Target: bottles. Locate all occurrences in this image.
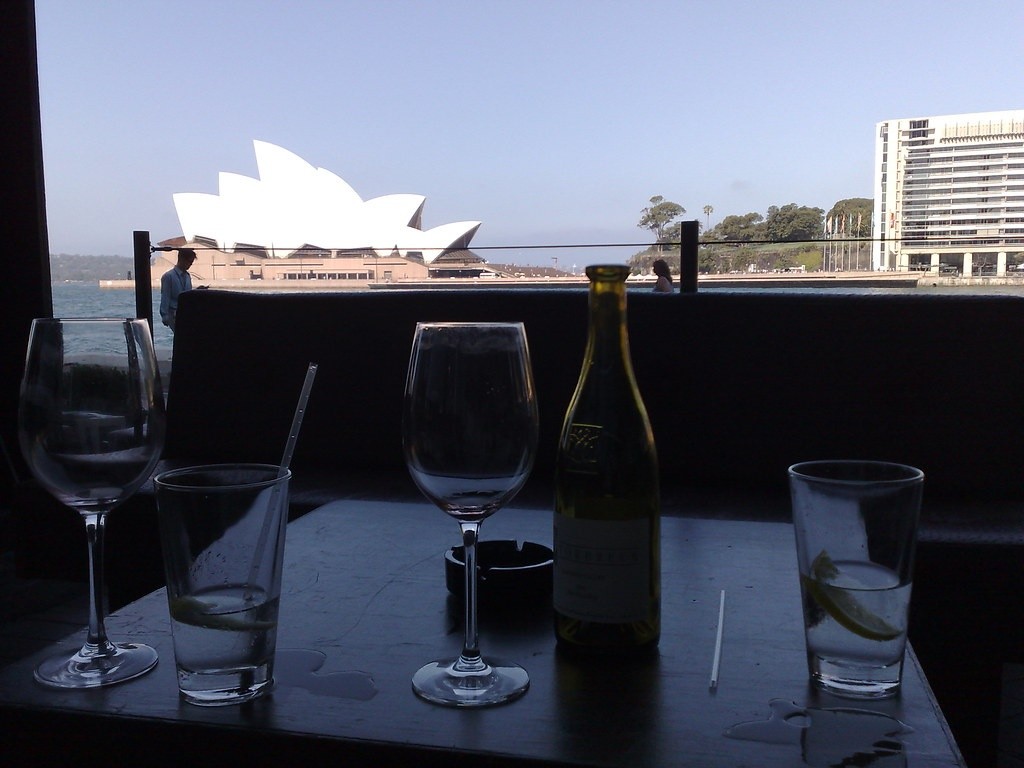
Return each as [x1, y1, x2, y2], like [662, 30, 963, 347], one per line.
[556, 264, 663, 664]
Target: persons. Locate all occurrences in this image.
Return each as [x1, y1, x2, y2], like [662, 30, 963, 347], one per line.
[652, 259, 675, 295]
[159, 250, 209, 336]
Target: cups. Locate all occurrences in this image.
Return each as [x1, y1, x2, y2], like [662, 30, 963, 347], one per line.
[788, 459, 928, 700]
[154, 461, 293, 707]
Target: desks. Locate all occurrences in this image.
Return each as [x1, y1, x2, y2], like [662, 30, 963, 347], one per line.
[0, 497, 967, 767]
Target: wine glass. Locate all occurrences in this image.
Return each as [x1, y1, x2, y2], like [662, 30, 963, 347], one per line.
[401, 320, 540, 709]
[21, 317, 166, 691]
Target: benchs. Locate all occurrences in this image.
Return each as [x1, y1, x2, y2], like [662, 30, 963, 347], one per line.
[106, 294, 1023, 665]
